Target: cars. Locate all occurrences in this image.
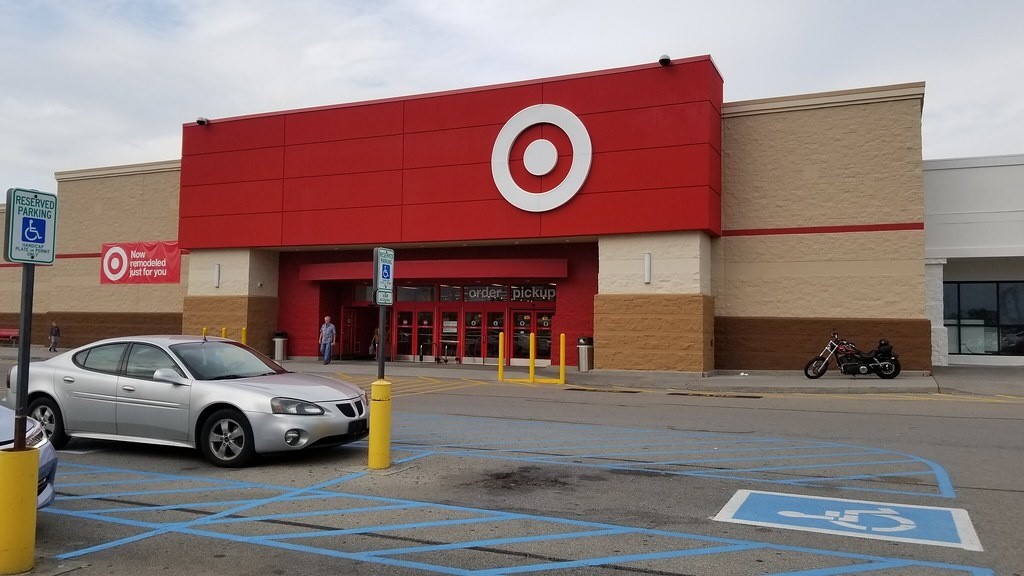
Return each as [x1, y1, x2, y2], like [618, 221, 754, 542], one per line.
[0, 404, 58, 512]
[5, 333, 372, 469]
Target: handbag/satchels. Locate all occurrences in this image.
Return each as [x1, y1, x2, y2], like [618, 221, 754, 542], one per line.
[369, 344, 374, 355]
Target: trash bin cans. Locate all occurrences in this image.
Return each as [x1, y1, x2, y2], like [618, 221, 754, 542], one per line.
[272, 331, 288, 361]
[577, 336, 594, 372]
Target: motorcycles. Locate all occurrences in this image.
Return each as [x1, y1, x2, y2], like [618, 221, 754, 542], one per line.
[804, 326, 902, 380]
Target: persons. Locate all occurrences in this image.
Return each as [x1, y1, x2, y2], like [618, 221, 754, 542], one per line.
[319, 316, 336, 365]
[49, 321, 60, 352]
[371, 327, 379, 359]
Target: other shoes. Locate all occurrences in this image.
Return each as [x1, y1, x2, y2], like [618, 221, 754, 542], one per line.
[324, 356, 332, 365]
[53, 349, 57, 352]
[49, 349, 51, 352]
